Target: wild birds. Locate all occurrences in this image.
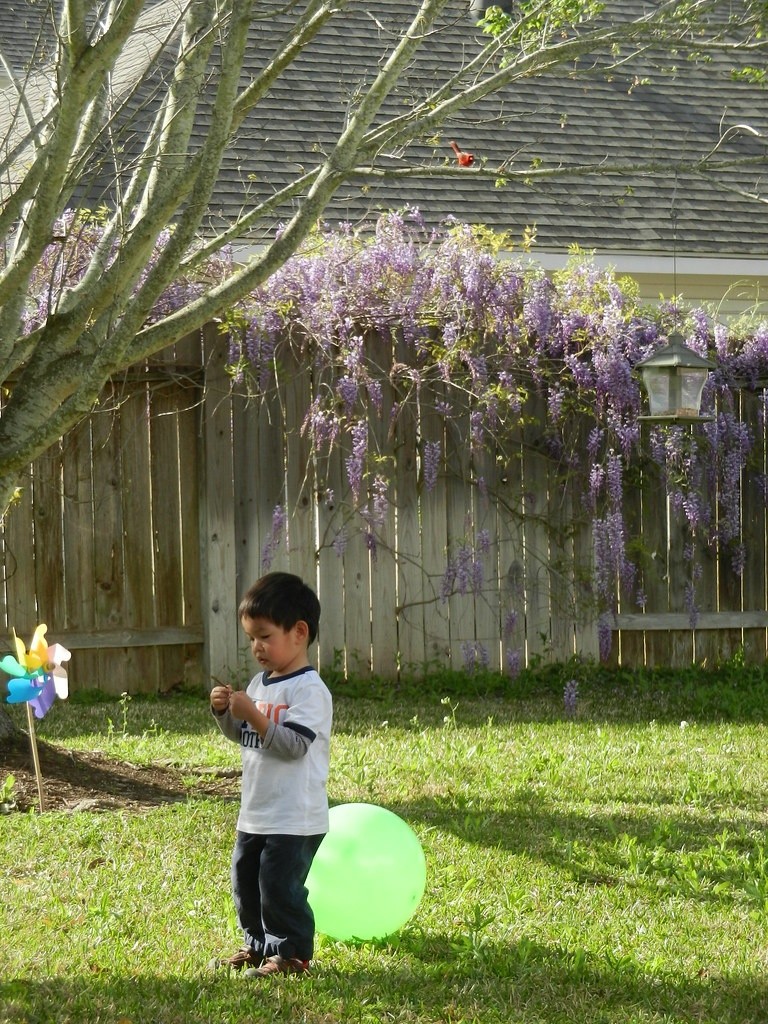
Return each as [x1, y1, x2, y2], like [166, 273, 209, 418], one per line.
[447, 141, 474, 168]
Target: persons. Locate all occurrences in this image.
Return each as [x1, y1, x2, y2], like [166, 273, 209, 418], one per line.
[209, 571, 336, 979]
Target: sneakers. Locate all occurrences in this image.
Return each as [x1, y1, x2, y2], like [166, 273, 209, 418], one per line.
[218, 944, 268, 971]
[245, 954, 308, 978]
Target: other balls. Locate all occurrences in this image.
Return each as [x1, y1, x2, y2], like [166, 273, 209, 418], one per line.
[307, 802, 428, 942]
